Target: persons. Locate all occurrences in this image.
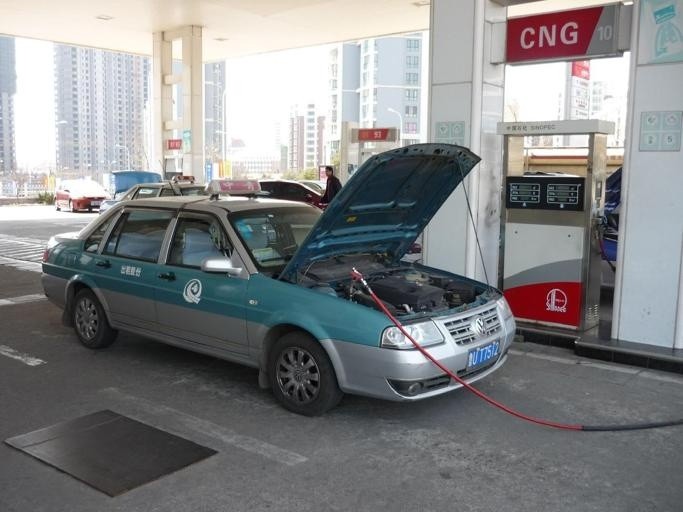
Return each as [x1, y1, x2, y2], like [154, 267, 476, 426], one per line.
[320, 166, 342, 203]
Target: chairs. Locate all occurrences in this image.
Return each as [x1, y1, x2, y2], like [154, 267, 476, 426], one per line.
[242, 230, 267, 252]
[169, 223, 223, 269]
